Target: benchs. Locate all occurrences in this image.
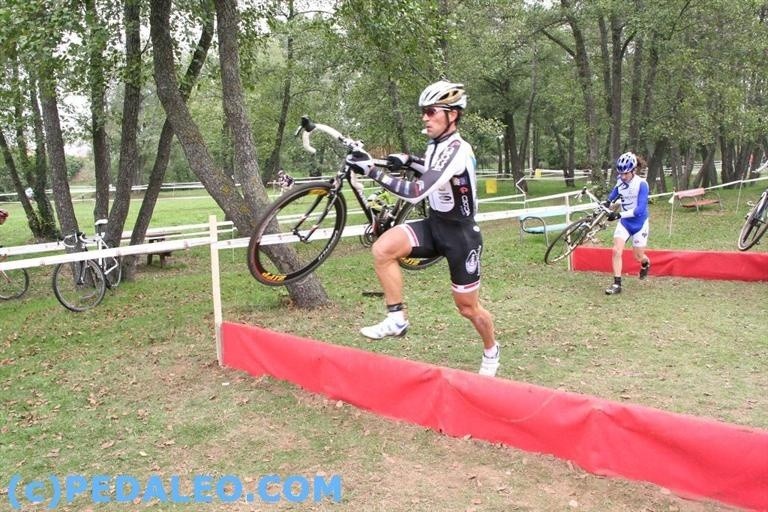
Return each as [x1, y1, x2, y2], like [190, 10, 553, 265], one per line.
[676, 185, 725, 217]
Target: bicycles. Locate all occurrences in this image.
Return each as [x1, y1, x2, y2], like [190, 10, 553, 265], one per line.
[245, 115, 449, 287]
[738, 161, 768, 251]
[54, 219, 124, 312]
[541, 186, 620, 266]
[0, 246, 29, 302]
[273, 182, 303, 205]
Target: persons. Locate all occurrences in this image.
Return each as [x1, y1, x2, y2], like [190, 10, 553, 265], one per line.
[605, 150, 652, 296]
[277, 170, 295, 195]
[344, 77, 503, 378]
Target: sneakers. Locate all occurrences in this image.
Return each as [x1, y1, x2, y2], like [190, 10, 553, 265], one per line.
[605, 282, 621, 294]
[359, 310, 408, 341]
[477, 344, 501, 378]
[638, 260, 650, 280]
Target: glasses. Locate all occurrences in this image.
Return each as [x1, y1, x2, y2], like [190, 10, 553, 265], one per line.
[421, 106, 452, 115]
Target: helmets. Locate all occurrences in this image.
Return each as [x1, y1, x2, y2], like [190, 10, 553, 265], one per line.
[616, 151, 638, 173]
[278, 169, 284, 176]
[417, 79, 466, 109]
